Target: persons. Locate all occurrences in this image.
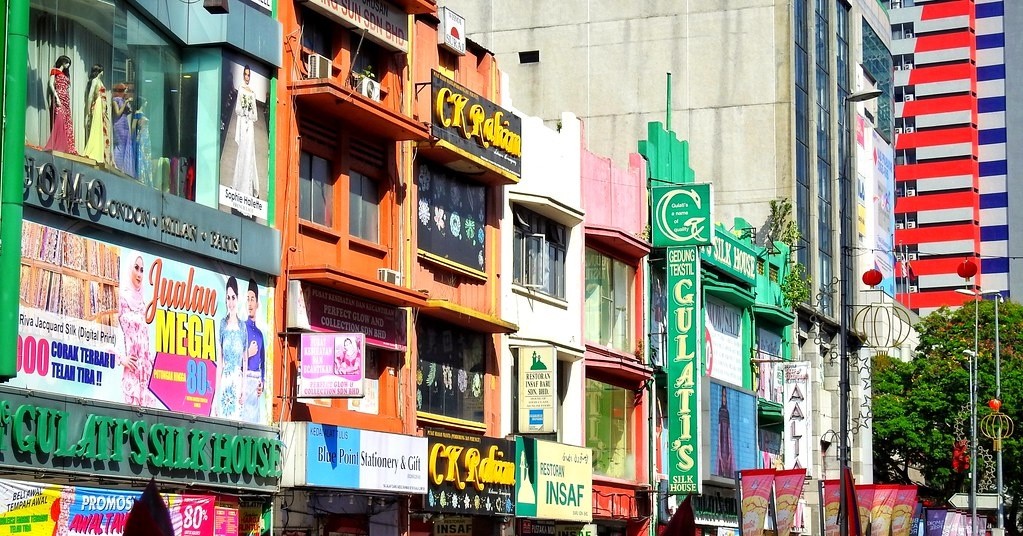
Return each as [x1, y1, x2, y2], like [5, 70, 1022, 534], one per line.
[131, 95, 154, 187]
[112, 82, 136, 176]
[716, 386, 737, 478]
[336, 338, 360, 376]
[213, 276, 247, 421]
[48, 54, 76, 154]
[234, 64, 259, 198]
[86, 64, 114, 164]
[104, 253, 161, 409]
[242, 278, 266, 425]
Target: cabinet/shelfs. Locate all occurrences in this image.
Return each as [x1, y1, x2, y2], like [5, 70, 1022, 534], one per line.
[17, 217, 120, 329]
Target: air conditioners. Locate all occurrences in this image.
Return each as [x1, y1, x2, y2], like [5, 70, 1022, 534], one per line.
[896, 253, 917, 261]
[907, 190, 916, 197]
[308, 54, 333, 79]
[906, 127, 915, 133]
[908, 286, 918, 293]
[895, 66, 901, 71]
[895, 128, 902, 134]
[895, 223, 905, 230]
[905, 94, 914, 102]
[907, 221, 916, 229]
[906, 33, 913, 39]
[377, 267, 403, 287]
[904, 64, 913, 70]
[356, 75, 381, 103]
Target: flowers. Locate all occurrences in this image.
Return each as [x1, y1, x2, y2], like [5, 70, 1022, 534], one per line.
[240, 93, 255, 115]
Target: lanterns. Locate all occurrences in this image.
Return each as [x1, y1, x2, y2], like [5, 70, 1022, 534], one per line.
[957, 260, 978, 281]
[862, 269, 882, 289]
[989, 399, 1001, 411]
